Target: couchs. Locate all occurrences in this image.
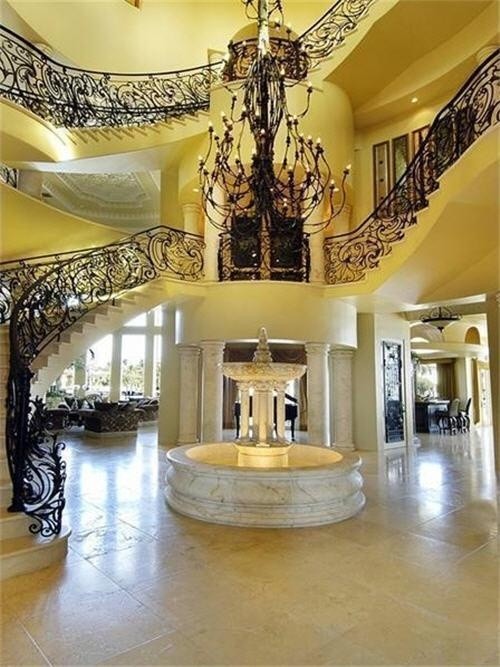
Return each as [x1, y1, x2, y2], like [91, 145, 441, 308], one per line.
[45, 390, 159, 439]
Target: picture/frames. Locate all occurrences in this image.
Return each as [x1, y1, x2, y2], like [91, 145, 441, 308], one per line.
[369, 101, 476, 221]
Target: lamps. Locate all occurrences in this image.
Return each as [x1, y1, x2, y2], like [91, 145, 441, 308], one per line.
[184, 1, 353, 239]
[418, 306, 463, 334]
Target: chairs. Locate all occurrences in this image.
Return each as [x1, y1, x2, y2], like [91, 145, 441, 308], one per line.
[435, 398, 471, 435]
